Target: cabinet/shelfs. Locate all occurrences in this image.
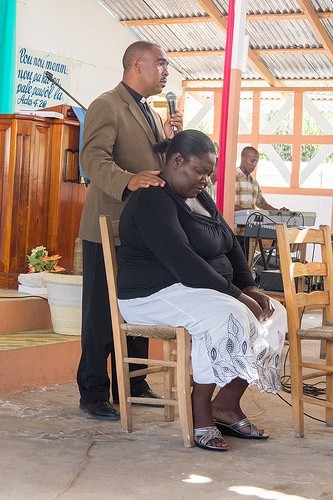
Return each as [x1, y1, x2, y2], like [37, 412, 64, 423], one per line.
[0, 114, 91, 288]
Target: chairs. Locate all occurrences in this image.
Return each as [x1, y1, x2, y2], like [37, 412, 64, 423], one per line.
[99, 214, 196, 448]
[275, 223, 333, 439]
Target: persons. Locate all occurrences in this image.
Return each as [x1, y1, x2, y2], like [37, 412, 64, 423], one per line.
[76, 41, 183, 421]
[202, 147, 283, 264]
[117, 129, 287, 451]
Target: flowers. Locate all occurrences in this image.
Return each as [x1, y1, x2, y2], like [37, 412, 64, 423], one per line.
[27, 245, 66, 274]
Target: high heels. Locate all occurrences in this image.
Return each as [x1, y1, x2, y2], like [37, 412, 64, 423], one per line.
[214, 418, 270, 440]
[192, 425, 230, 452]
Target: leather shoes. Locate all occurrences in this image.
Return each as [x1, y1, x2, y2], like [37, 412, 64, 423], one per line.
[112, 389, 166, 408]
[79, 400, 121, 421]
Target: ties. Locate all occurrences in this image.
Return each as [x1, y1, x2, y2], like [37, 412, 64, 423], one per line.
[142, 103, 161, 141]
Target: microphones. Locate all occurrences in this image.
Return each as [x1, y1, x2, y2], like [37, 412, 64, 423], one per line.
[166, 91, 179, 131]
